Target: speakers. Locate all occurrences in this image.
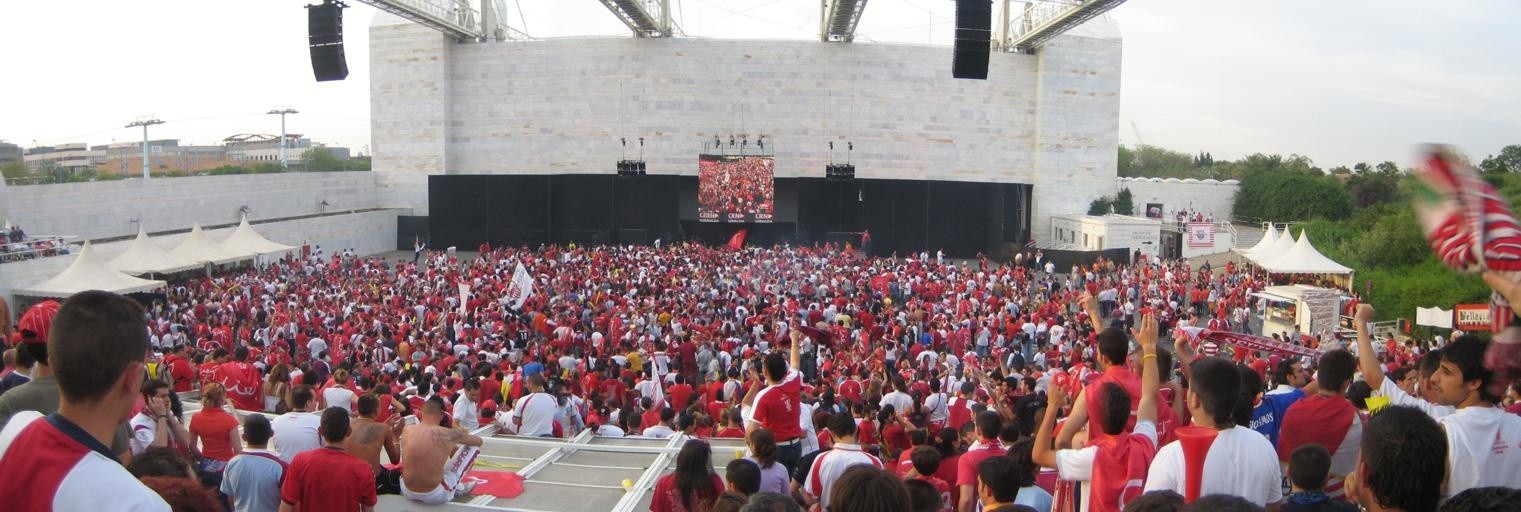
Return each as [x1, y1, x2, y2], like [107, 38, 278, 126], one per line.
[308, 5, 349, 82]
[952, 0, 993, 78]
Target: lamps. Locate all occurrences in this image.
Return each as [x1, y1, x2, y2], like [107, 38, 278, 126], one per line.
[639, 137, 644, 161]
[848, 140, 853, 164]
[714, 133, 766, 157]
[621, 138, 626, 160]
[829, 141, 834, 165]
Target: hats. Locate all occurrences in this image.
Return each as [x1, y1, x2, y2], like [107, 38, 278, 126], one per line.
[20, 300, 58, 345]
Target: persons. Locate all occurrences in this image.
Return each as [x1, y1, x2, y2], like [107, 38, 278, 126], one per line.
[698, 158, 774, 207]
[2, 209, 1520, 510]
